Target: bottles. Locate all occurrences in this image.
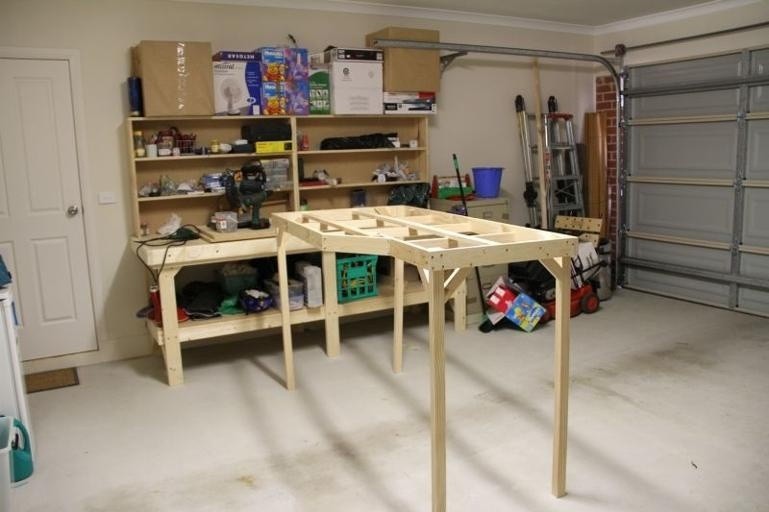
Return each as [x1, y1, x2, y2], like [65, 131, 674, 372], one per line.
[134, 131, 147, 157]
[211, 136, 220, 153]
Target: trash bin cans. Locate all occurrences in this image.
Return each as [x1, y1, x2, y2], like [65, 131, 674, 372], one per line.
[594, 238, 613, 301]
[0, 415, 14, 512]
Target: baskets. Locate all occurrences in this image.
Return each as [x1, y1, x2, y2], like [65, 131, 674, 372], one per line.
[335, 253, 382, 306]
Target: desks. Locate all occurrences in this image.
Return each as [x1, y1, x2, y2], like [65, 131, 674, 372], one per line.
[132, 238, 468, 387]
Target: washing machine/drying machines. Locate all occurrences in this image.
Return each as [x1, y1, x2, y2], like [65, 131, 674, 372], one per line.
[430, 173, 509, 320]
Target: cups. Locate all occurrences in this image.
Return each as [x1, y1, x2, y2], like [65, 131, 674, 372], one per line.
[146, 144, 158, 157]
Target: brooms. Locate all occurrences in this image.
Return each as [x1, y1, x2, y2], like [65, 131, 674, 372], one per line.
[452, 152, 509, 332]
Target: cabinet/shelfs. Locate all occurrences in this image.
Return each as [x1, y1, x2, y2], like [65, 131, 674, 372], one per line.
[126, 114, 430, 238]
[432, 196, 512, 318]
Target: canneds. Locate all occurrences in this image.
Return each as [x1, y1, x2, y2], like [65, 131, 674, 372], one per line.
[210, 140, 219, 153]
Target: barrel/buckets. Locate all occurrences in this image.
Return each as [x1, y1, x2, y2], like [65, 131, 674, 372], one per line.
[472, 167, 506, 197]
[293, 260, 322, 307]
[0, 414, 35, 482]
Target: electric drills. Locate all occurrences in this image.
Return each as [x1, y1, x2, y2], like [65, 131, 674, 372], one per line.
[243, 190, 274, 229]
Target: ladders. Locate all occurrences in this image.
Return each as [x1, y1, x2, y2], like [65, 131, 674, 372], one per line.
[514, 94, 587, 232]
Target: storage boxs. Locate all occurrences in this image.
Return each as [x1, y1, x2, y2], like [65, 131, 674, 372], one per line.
[308, 46, 384, 114]
[306, 61, 331, 113]
[484, 275, 546, 332]
[213, 49, 262, 115]
[130, 26, 214, 117]
[364, 27, 440, 112]
[256, 45, 309, 115]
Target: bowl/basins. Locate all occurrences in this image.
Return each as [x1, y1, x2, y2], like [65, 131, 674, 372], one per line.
[238, 296, 273, 313]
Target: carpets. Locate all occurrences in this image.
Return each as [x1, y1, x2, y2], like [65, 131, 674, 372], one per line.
[24, 366, 79, 394]
